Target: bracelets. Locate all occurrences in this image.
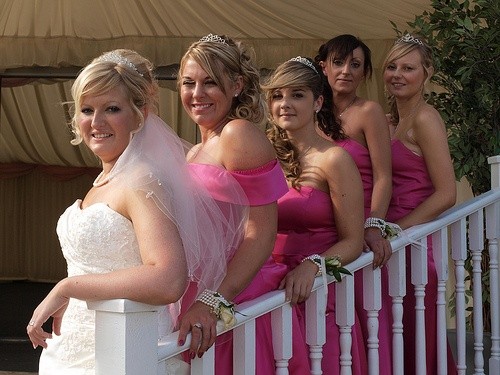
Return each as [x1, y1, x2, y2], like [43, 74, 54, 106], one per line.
[363, 217, 402, 238]
[300, 253, 353, 284]
[195, 287, 249, 330]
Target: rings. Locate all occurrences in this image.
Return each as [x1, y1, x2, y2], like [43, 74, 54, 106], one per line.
[192, 323, 203, 330]
[29, 320, 35, 326]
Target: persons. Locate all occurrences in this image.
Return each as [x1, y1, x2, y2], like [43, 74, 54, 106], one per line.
[24, 47, 249, 375]
[381, 33, 458, 375]
[171, 31, 308, 375]
[263, 57, 368, 375]
[315, 34, 393, 375]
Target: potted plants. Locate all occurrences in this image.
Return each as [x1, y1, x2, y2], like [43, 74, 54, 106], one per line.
[390, 0, 500, 375]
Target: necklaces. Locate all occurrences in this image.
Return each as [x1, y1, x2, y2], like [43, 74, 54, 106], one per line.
[91, 152, 148, 189]
[336, 95, 359, 120]
[297, 135, 319, 159]
